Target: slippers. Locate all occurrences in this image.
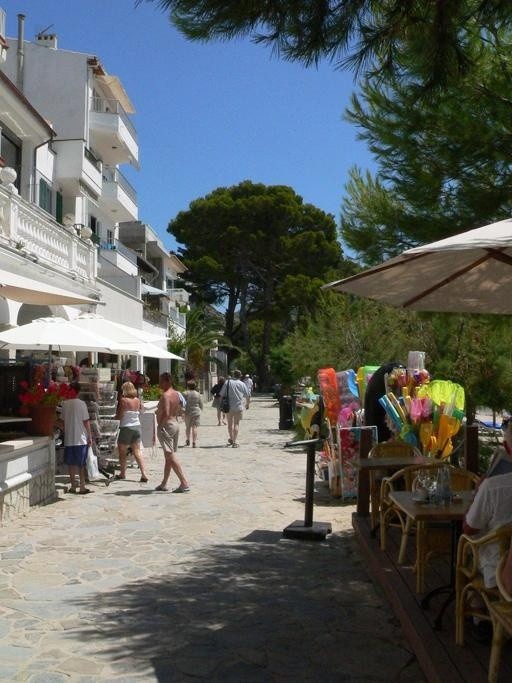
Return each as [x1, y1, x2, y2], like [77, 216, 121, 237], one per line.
[64, 474, 193, 500]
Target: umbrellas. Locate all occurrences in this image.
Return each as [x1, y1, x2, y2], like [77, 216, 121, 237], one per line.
[319, 217, 512, 315]
[0, 310, 186, 366]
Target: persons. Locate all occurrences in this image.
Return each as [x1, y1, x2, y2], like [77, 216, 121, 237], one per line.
[181, 379, 204, 448]
[154, 372, 190, 493]
[210, 369, 257, 448]
[461, 417, 512, 614]
[60, 381, 94, 494]
[112, 381, 149, 482]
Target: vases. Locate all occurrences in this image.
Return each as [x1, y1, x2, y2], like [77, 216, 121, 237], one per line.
[26, 406, 58, 436]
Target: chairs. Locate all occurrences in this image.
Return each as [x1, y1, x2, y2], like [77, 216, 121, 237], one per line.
[365, 439, 511, 682]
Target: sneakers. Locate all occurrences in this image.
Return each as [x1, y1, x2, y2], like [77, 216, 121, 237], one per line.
[227, 438, 240, 448]
[185, 437, 198, 449]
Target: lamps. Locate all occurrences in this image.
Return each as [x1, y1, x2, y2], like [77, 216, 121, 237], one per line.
[73, 222, 93, 239]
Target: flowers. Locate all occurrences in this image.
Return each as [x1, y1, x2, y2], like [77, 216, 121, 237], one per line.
[18, 379, 79, 416]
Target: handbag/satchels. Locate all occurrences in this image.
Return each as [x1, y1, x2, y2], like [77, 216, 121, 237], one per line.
[218, 399, 232, 414]
[85, 447, 104, 482]
